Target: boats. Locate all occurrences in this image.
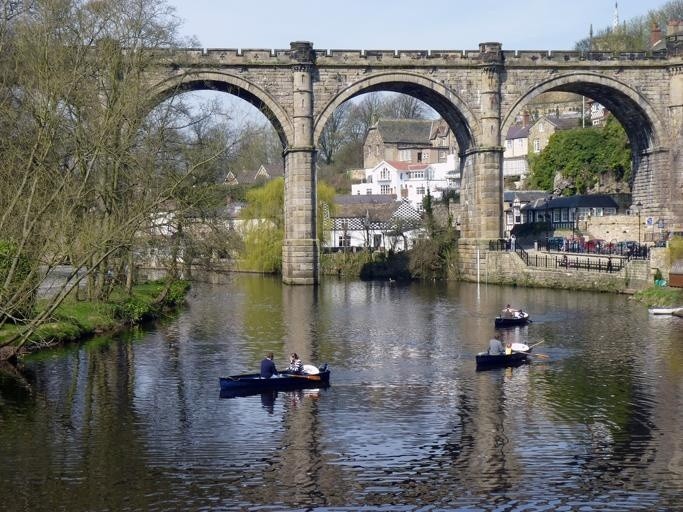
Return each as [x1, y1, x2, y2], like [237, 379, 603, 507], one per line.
[475, 342, 531, 368]
[218, 363, 331, 392]
[495, 312, 529, 329]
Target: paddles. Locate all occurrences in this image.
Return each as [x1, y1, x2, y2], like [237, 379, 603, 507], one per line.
[514, 350, 549, 360]
[279, 373, 320, 381]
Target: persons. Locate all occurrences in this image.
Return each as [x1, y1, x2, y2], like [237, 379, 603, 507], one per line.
[260, 352, 287, 378]
[260, 391, 278, 416]
[510, 234, 516, 250]
[486, 333, 505, 355]
[559, 233, 586, 253]
[285, 390, 303, 408]
[501, 304, 521, 319]
[288, 352, 303, 375]
[489, 367, 505, 388]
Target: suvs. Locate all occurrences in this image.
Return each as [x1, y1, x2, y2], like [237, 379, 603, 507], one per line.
[546, 236, 564, 251]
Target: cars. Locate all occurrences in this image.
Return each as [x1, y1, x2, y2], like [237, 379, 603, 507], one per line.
[581, 239, 642, 257]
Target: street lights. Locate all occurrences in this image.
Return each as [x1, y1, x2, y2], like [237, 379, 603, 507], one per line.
[636, 201, 643, 257]
